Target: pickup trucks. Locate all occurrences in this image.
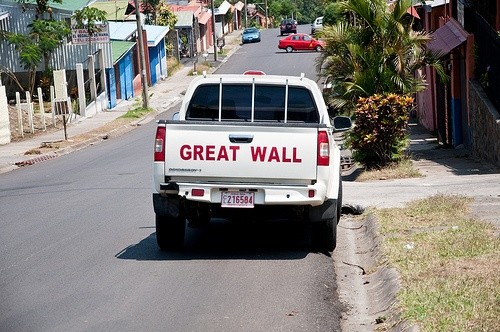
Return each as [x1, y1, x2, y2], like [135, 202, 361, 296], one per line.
[152, 70, 352, 253]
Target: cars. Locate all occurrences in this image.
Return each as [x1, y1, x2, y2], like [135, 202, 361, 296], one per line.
[310, 16, 324, 35]
[277, 33, 326, 53]
[241, 27, 261, 42]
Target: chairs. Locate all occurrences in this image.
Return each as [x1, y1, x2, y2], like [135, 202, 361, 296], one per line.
[272, 101, 307, 122]
[292, 36, 304, 40]
[208, 99, 238, 119]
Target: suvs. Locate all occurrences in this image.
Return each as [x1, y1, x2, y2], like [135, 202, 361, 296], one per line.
[279, 18, 301, 34]
